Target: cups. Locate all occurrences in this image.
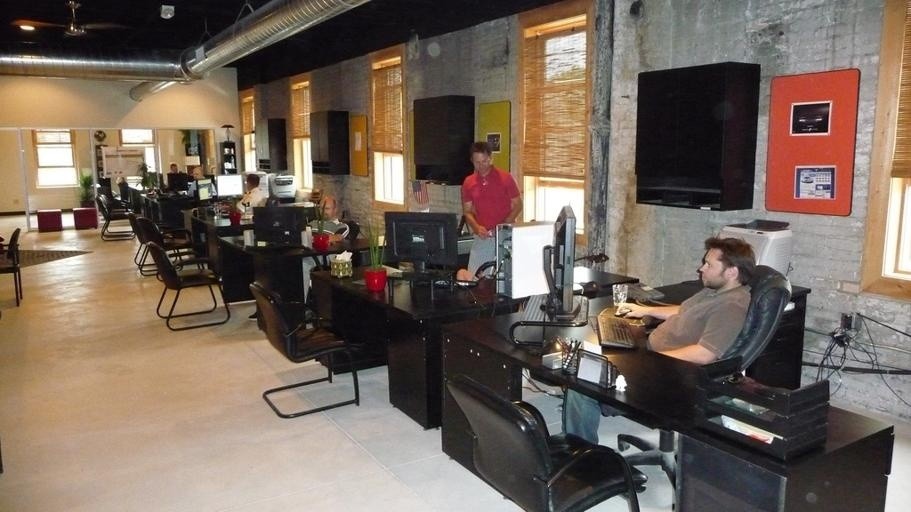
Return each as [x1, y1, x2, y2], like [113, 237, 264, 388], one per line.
[562, 349, 577, 375]
[612, 285, 628, 313]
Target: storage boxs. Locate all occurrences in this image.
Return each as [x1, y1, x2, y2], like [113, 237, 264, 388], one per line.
[36, 208, 63, 232]
[72, 207, 99, 230]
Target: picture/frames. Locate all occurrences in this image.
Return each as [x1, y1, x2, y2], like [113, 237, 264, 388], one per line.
[789, 100, 832, 136]
[792, 163, 837, 201]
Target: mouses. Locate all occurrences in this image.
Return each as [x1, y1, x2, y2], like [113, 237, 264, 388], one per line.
[615, 308, 631, 317]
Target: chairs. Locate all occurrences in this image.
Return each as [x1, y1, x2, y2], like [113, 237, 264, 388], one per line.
[341, 218, 362, 243]
[615, 263, 791, 511]
[95, 192, 138, 242]
[145, 240, 232, 332]
[446, 371, 647, 511]
[0, 227, 25, 309]
[134, 216, 191, 277]
[95, 183, 136, 234]
[249, 277, 360, 419]
[128, 212, 178, 267]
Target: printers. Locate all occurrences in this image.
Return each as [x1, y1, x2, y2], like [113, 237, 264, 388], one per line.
[269, 174, 297, 198]
[717, 219, 794, 278]
[241, 171, 268, 196]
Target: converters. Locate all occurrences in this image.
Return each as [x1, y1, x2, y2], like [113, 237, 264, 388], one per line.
[841, 312, 851, 330]
[830, 331, 851, 347]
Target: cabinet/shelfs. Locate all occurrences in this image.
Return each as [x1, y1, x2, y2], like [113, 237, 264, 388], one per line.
[635, 59, 763, 212]
[219, 141, 238, 174]
[411, 95, 475, 188]
[308, 111, 351, 176]
[253, 117, 288, 173]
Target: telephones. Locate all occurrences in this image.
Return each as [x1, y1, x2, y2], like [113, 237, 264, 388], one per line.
[627, 282, 664, 303]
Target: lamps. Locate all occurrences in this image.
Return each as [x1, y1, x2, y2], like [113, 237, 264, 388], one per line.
[221, 124, 235, 141]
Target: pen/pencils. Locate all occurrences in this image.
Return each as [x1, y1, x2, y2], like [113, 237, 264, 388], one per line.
[556, 335, 581, 370]
[493, 260, 504, 277]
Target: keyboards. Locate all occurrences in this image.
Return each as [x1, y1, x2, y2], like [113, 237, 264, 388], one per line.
[387, 271, 403, 279]
[597, 315, 636, 350]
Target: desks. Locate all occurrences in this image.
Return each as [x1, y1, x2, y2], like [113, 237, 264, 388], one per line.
[212, 230, 376, 320]
[307, 257, 522, 433]
[178, 203, 254, 275]
[435, 285, 897, 512]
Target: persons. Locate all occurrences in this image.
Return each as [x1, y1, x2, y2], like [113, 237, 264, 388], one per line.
[460, 142, 523, 276]
[239, 175, 266, 219]
[562, 238, 757, 445]
[166, 163, 179, 186]
[247, 195, 350, 320]
[184, 167, 217, 231]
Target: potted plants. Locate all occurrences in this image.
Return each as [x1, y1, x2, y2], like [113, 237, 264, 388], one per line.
[306, 200, 329, 252]
[78, 173, 95, 208]
[229, 198, 241, 225]
[359, 218, 387, 293]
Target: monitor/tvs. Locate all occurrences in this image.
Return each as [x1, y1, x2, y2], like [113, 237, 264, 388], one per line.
[216, 174, 244, 203]
[543, 206, 581, 320]
[158, 172, 164, 192]
[167, 173, 188, 196]
[196, 178, 214, 208]
[384, 210, 458, 281]
[253, 206, 315, 250]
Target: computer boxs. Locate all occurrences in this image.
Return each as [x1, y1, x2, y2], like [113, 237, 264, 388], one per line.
[495, 220, 555, 300]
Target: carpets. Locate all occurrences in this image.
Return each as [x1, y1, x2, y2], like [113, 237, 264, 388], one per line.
[18, 249, 94, 268]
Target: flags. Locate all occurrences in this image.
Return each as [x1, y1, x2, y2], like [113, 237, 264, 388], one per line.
[412, 180, 429, 207]
